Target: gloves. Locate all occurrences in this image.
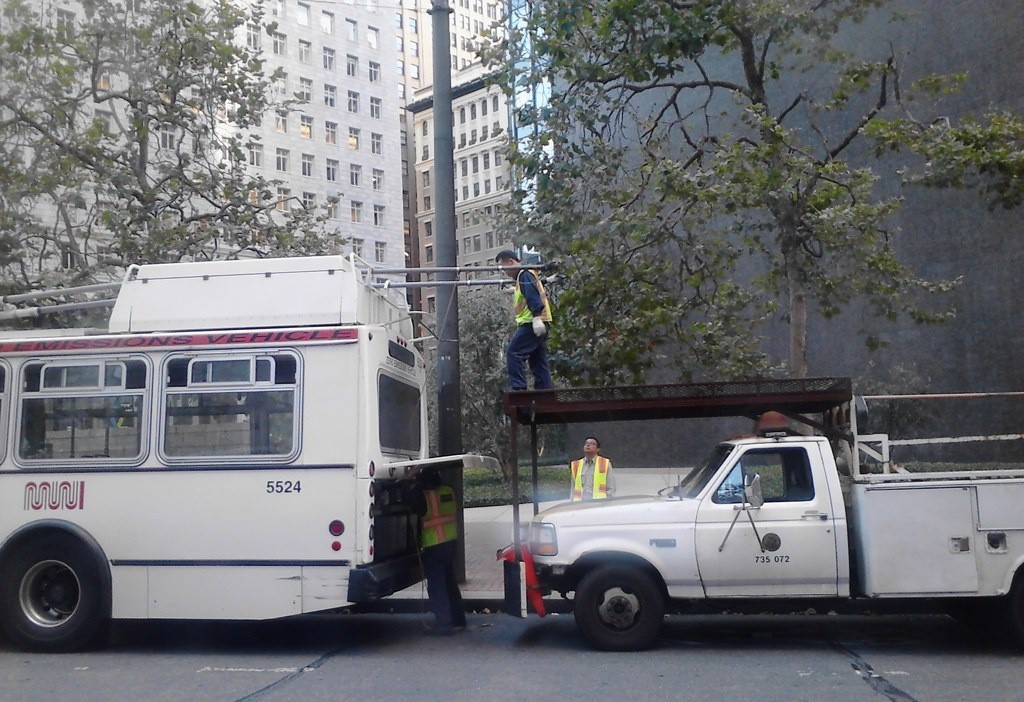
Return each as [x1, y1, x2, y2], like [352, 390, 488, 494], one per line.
[533, 317, 547, 336]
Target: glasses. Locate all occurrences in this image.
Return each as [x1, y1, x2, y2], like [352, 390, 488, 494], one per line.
[584, 442, 598, 447]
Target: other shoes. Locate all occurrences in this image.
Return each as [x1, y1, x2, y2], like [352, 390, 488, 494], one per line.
[428, 626, 463, 635]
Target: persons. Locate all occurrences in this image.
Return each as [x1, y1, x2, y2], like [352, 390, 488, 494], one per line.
[569, 438, 617, 501]
[494, 249, 561, 390]
[404, 463, 469, 637]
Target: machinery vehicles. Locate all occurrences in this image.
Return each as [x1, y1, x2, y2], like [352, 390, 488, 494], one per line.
[0, 256, 502, 646]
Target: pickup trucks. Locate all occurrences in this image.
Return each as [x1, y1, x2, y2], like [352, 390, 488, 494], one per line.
[521, 426, 1023, 655]
